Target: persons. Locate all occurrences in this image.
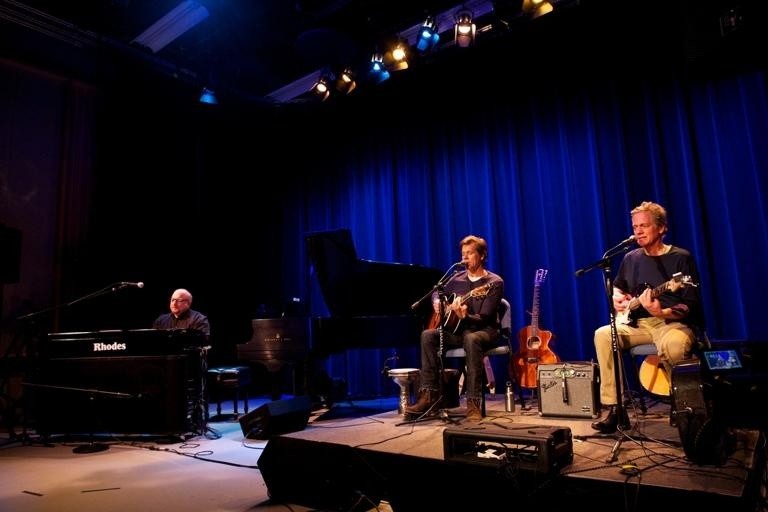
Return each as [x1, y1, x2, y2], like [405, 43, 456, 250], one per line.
[592, 201, 707, 433]
[153, 288, 211, 341]
[405, 235, 505, 421]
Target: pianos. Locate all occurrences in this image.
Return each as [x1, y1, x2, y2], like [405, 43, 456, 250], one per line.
[236, 228, 450, 409]
[24, 329, 210, 438]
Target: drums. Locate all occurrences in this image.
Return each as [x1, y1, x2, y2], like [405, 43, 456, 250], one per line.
[444, 370, 460, 408]
[388, 368, 420, 414]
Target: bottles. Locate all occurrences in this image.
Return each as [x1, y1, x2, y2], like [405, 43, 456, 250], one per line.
[504, 381, 515, 412]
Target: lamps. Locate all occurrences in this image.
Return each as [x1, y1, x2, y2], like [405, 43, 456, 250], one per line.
[453, 8, 478, 50]
[522, 0, 555, 20]
[309, 76, 333, 102]
[337, 63, 359, 94]
[417, 15, 440, 52]
[386, 37, 410, 71]
[369, 53, 390, 82]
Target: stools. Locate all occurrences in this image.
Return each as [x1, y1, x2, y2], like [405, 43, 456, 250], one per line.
[204, 365, 250, 420]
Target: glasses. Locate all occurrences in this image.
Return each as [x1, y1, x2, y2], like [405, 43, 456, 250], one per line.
[171, 298, 193, 303]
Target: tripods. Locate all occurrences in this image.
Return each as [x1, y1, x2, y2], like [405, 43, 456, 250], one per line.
[395, 311, 466, 427]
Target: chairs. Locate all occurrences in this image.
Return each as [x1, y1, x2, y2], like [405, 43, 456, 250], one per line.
[446, 298, 526, 417]
[627, 330, 711, 415]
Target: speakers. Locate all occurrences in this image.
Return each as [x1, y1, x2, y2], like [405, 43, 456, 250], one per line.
[237, 398, 311, 439]
[536, 362, 600, 418]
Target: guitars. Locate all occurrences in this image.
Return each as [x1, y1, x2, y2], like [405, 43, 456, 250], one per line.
[429, 281, 497, 334]
[508, 269, 561, 401]
[639, 355, 673, 400]
[613, 272, 699, 327]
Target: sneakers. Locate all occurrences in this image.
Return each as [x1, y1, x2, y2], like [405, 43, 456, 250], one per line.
[465, 396, 485, 422]
[404, 391, 440, 414]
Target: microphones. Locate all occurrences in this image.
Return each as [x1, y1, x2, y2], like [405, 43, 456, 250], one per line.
[616, 234, 638, 249]
[120, 281, 145, 289]
[455, 261, 467, 266]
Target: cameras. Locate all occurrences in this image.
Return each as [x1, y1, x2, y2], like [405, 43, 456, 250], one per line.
[570, 271, 682, 465]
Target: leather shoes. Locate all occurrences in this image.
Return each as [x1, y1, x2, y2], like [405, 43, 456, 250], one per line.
[591, 405, 632, 432]
